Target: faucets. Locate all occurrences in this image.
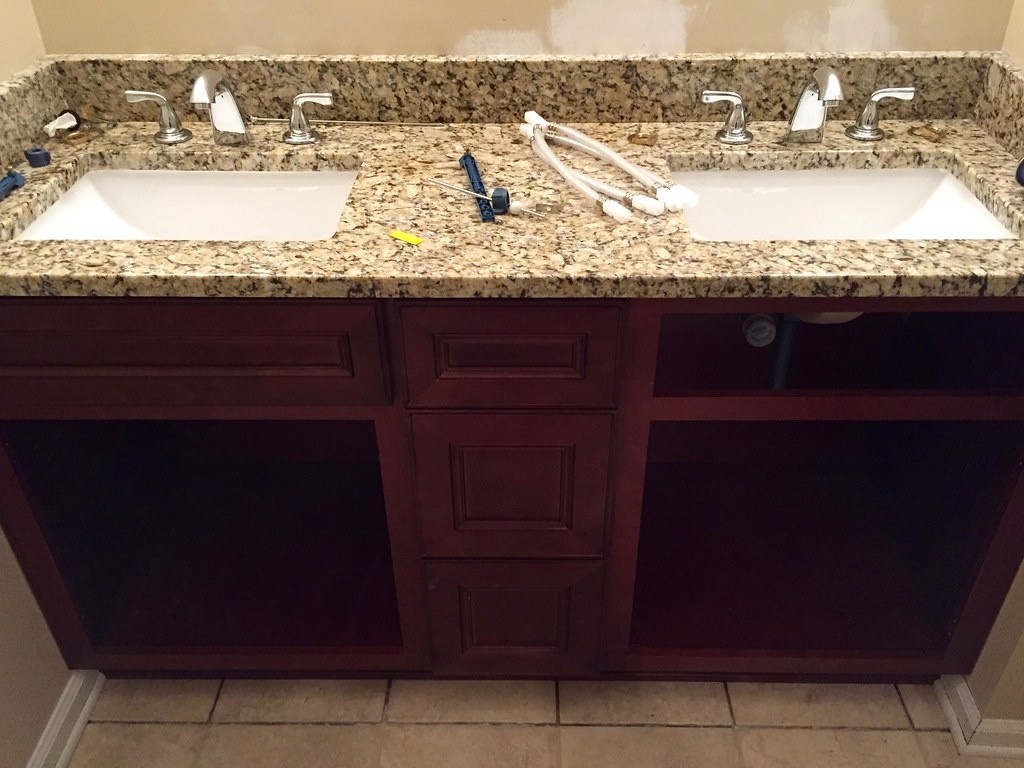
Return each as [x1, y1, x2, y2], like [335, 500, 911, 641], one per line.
[189, 69, 253, 146]
[784, 66, 844, 143]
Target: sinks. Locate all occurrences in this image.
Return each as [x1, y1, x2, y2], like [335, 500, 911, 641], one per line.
[3, 150, 364, 241]
[667, 150, 1024, 241]
